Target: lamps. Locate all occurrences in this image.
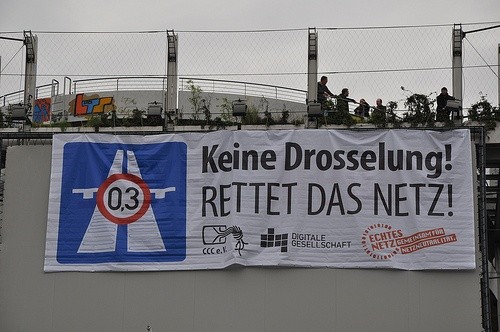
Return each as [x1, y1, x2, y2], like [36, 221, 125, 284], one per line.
[446, 99, 463, 121]
[147, 101, 162, 126]
[306, 103, 324, 127]
[9, 103, 32, 126]
[232, 99, 248, 129]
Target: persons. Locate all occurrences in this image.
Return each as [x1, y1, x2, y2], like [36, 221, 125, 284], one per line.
[354, 99, 370, 118]
[336, 87, 356, 113]
[317, 76, 336, 104]
[373, 97, 386, 115]
[437, 86, 456, 116]
